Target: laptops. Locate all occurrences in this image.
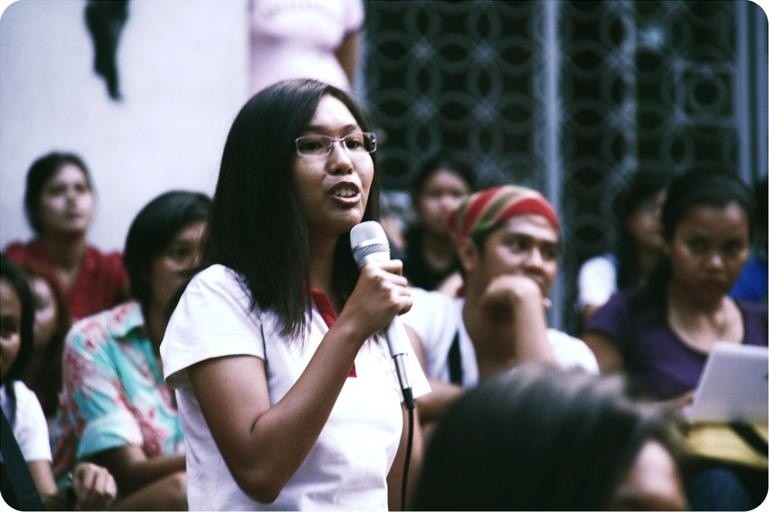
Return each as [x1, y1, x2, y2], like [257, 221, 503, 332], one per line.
[680, 340, 768, 424]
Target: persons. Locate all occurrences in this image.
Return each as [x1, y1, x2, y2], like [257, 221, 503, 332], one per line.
[5, 151, 132, 323]
[401, 157, 476, 291]
[249, 0, 367, 102]
[0, 252, 117, 510]
[20, 258, 74, 432]
[400, 185, 599, 443]
[159, 78, 431, 511]
[412, 356, 692, 511]
[51, 188, 212, 511]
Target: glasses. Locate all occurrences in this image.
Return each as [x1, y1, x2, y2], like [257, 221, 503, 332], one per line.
[294, 131, 377, 158]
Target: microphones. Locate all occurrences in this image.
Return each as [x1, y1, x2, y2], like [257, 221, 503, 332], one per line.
[350, 221, 414, 409]
